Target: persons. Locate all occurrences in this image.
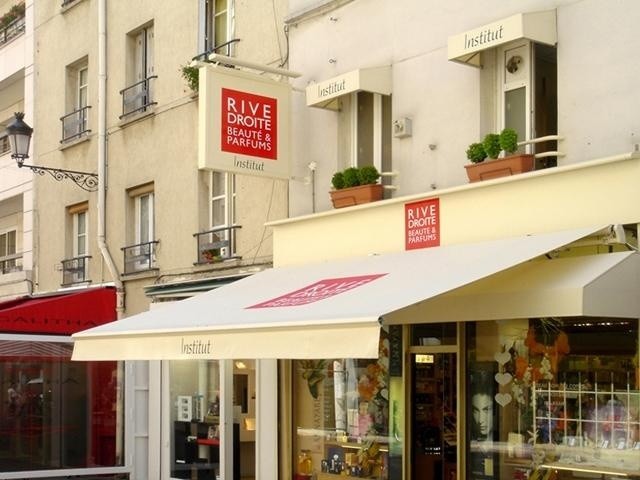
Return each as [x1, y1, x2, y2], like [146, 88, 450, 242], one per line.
[466, 381, 495, 474]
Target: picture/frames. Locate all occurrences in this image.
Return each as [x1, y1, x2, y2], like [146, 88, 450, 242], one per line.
[466, 360, 500, 479]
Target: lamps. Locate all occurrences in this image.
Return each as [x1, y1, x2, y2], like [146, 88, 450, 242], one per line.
[6, 112, 101, 194]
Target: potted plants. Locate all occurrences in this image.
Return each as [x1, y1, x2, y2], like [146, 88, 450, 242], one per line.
[464, 128, 535, 183]
[328, 164, 384, 209]
